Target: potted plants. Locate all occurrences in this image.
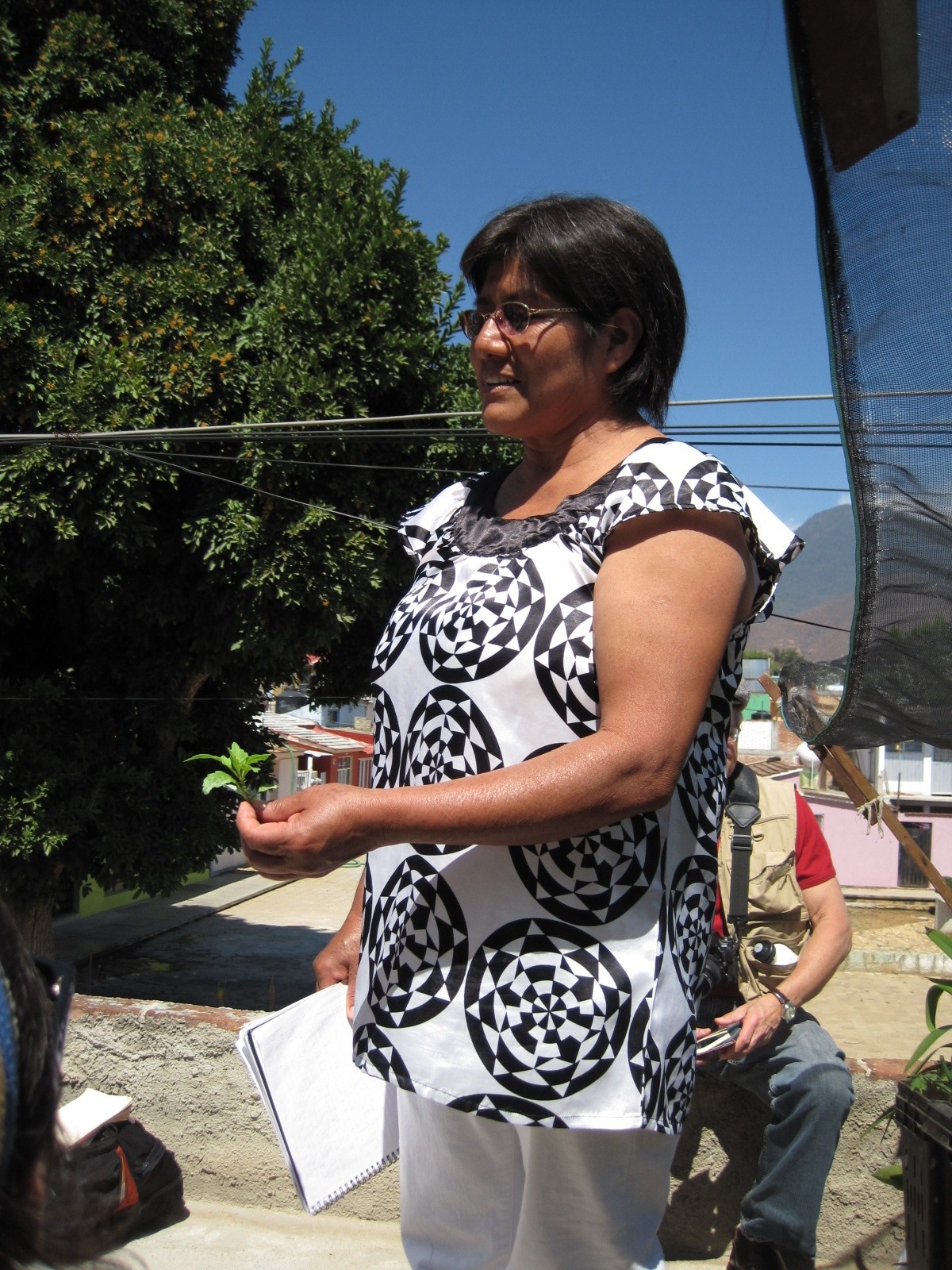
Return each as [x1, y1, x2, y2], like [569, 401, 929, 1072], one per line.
[856, 922, 952, 1270]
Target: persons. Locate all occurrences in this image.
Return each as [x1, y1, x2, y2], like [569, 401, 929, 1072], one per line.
[234, 194, 803, 1270]
[693, 680, 855, 1270]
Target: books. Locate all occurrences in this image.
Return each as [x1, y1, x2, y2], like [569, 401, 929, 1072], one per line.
[234, 977, 401, 1215]
[696, 1020, 740, 1057]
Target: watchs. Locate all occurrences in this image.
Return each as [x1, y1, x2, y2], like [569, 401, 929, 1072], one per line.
[768, 987, 798, 1024]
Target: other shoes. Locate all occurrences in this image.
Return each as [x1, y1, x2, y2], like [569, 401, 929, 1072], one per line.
[727, 1225, 814, 1270]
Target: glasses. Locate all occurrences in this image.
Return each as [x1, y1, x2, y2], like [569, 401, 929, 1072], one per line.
[457, 303, 586, 339]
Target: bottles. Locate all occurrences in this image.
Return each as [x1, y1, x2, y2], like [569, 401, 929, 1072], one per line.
[753, 940, 798, 965]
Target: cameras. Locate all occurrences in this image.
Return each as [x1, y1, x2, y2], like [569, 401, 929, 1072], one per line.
[700, 935, 738, 996]
